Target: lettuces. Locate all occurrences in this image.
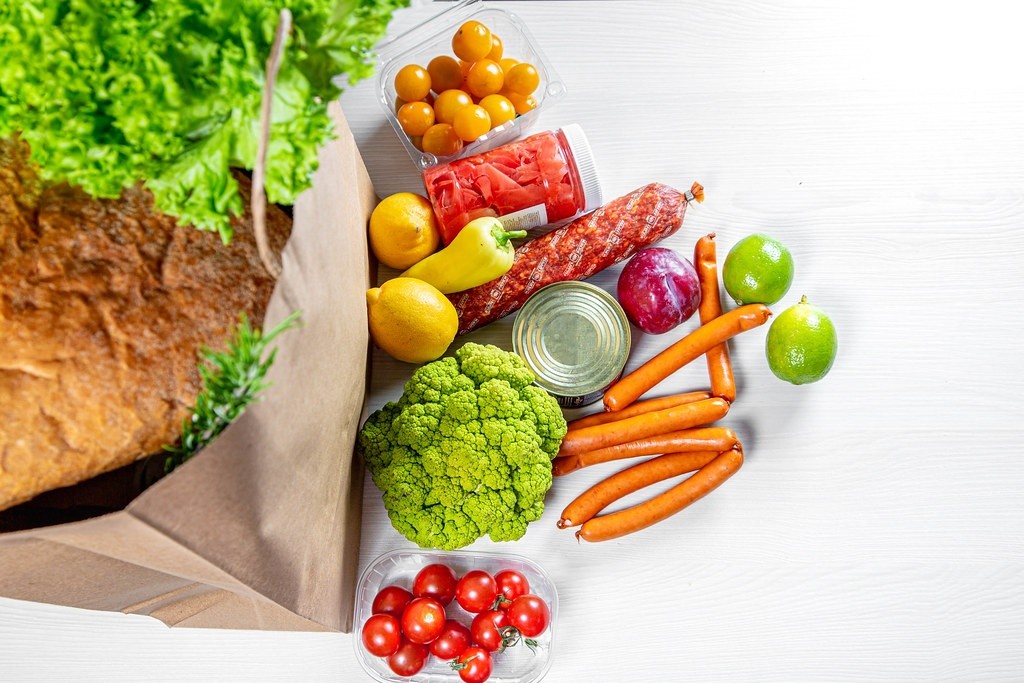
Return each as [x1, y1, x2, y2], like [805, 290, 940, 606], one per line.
[0, 2, 420, 247]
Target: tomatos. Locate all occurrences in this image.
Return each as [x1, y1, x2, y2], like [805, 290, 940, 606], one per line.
[365, 566, 553, 683]
[395, 21, 539, 157]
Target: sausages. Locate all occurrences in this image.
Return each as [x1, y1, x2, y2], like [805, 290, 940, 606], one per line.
[546, 230, 772, 544]
[444, 181, 713, 338]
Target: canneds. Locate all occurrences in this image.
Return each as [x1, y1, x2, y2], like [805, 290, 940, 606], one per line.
[423, 122, 612, 249]
[510, 279, 635, 410]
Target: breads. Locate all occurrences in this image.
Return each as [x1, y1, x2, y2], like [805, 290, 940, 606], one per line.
[0, 128, 294, 512]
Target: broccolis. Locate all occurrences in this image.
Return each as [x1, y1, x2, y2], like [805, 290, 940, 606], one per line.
[357, 340, 567, 551]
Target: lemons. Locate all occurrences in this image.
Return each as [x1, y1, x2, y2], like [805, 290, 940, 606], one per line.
[367, 189, 459, 367]
[725, 236, 838, 387]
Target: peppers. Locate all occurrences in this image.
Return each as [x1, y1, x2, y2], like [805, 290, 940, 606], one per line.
[395, 214, 533, 297]
